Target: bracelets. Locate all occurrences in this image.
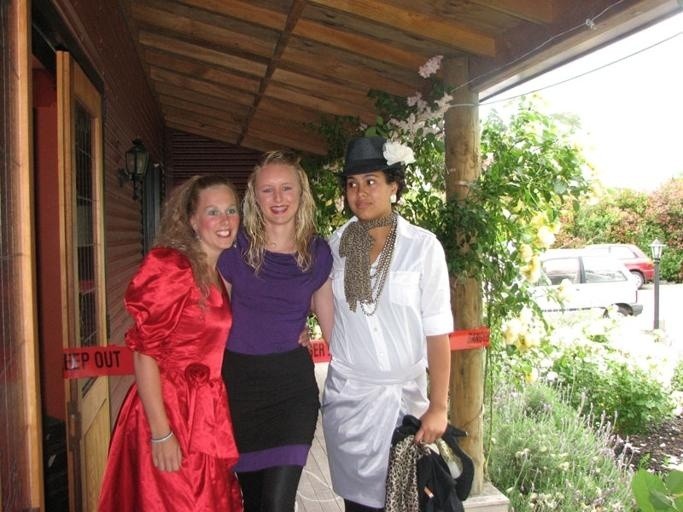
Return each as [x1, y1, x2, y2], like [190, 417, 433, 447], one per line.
[150, 431, 175, 444]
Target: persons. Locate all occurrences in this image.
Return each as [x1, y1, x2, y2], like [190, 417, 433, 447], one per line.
[215, 149, 334, 512]
[312, 137, 454, 512]
[98, 173, 245, 512]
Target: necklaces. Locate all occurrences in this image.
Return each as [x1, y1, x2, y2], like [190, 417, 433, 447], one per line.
[360, 214, 399, 317]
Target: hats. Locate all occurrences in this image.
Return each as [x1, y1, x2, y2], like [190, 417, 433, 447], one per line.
[332, 134, 403, 177]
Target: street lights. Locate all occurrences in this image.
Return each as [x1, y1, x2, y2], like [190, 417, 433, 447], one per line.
[648, 237, 666, 337]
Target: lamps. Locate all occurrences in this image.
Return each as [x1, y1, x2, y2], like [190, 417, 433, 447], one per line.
[118, 138, 149, 200]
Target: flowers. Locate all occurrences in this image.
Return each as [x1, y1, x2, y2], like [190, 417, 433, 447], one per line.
[382, 141, 416, 167]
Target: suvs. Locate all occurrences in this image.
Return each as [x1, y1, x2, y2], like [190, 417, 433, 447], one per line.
[504, 247, 643, 323]
[581, 243, 654, 293]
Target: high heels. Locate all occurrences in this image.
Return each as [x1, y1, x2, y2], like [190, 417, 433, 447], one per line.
[432, 419, 475, 503]
